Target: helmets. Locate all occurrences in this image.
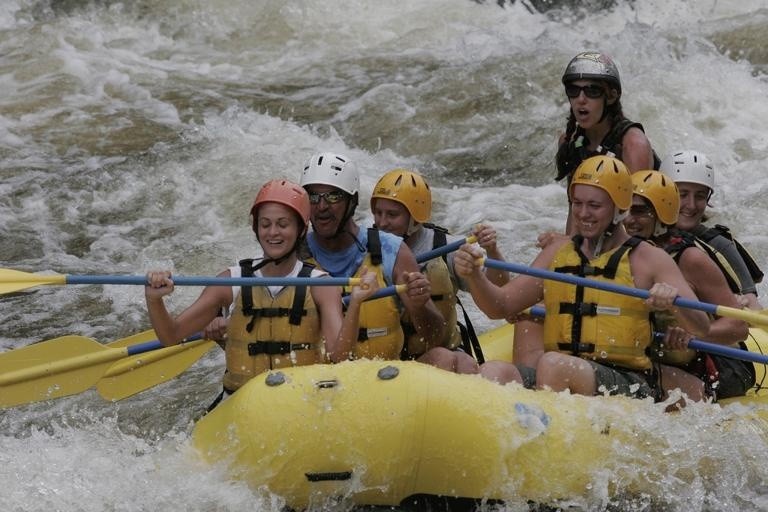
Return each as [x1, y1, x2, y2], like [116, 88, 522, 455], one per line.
[661, 149, 715, 189]
[562, 51, 621, 93]
[249, 181, 312, 228]
[567, 155, 633, 212]
[299, 153, 360, 196]
[371, 170, 434, 222]
[629, 169, 680, 224]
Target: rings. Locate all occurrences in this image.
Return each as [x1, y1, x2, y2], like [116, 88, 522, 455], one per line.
[676, 335, 683, 342]
[418, 286, 424, 293]
[487, 235, 491, 239]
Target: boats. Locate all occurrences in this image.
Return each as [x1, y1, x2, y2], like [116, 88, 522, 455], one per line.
[190, 323, 767, 511]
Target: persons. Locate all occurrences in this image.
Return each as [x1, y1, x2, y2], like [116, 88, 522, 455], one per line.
[536, 51, 663, 249]
[200, 152, 457, 374]
[624, 170, 750, 411]
[656, 148, 764, 400]
[453, 155, 711, 402]
[369, 169, 510, 377]
[143, 181, 381, 418]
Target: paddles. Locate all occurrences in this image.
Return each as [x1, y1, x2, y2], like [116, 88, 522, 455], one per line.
[0, 269, 368, 294]
[95, 237, 477, 402]
[0, 283, 409, 406]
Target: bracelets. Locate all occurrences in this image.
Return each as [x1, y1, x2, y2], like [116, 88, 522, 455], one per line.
[145, 295, 162, 303]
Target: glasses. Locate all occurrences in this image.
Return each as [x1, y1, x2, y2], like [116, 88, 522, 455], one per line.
[307, 191, 346, 205]
[566, 84, 603, 98]
